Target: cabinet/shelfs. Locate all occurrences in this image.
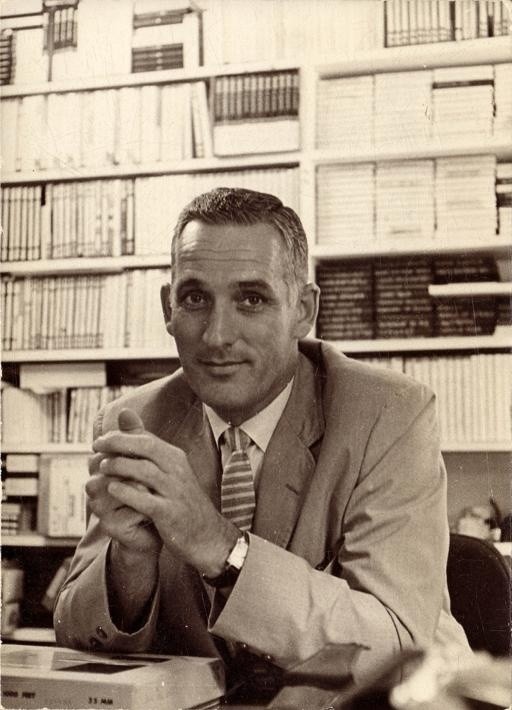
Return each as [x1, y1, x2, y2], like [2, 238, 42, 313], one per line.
[1, 2, 511, 649]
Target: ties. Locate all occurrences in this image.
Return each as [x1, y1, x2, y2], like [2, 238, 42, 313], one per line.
[219, 426, 256, 535]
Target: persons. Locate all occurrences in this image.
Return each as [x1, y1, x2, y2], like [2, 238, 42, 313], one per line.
[52, 187, 472, 710]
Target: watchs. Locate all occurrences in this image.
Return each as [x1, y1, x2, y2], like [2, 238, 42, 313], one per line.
[200, 532, 249, 589]
